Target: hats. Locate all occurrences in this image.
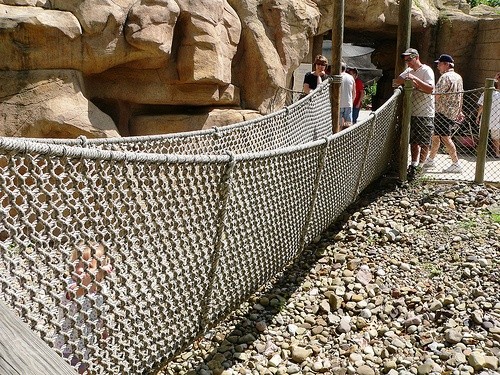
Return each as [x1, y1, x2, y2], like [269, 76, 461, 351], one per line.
[434, 54, 454, 62]
[347, 65, 358, 74]
[402, 48, 418, 55]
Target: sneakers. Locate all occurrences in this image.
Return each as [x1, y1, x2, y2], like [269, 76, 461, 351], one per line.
[407, 165, 424, 177]
[423, 159, 435, 168]
[442, 164, 462, 173]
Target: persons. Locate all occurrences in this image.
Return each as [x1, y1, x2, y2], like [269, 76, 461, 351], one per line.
[475, 72, 500, 159]
[394, 48, 436, 178]
[349, 68, 363, 126]
[424, 53, 464, 173]
[302, 54, 329, 96]
[340, 61, 356, 132]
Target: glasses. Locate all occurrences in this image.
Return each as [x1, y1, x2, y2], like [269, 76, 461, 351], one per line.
[316, 63, 325, 66]
[404, 57, 413, 62]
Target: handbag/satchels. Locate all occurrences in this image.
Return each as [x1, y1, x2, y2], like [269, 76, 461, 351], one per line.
[298, 94, 307, 100]
